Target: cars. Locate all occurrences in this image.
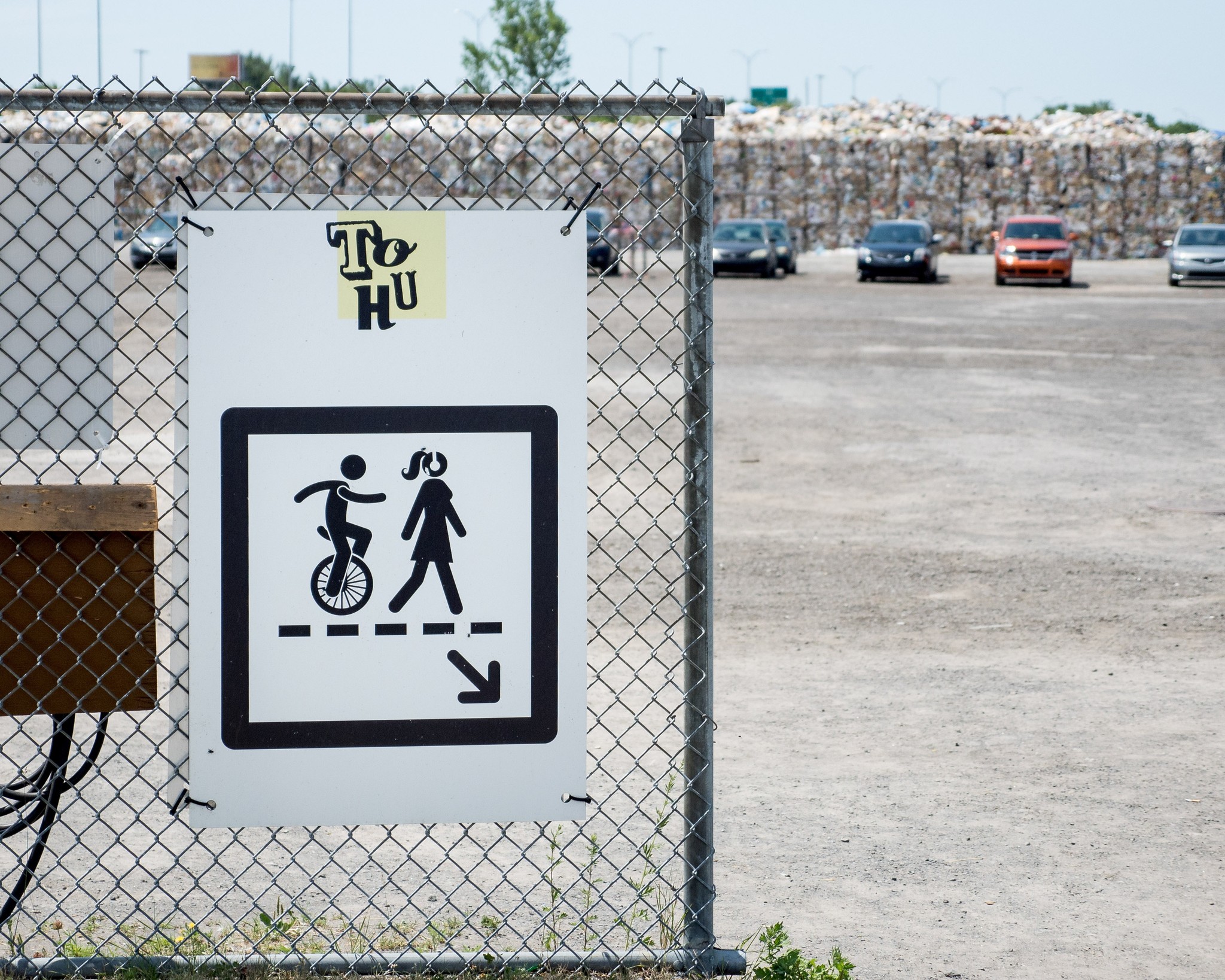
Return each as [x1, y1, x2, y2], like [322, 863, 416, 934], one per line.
[713, 218, 778, 278]
[990, 215, 1080, 288]
[130, 212, 178, 271]
[853, 221, 943, 284]
[587, 208, 621, 277]
[764, 218, 797, 275]
[1161, 222, 1225, 287]
